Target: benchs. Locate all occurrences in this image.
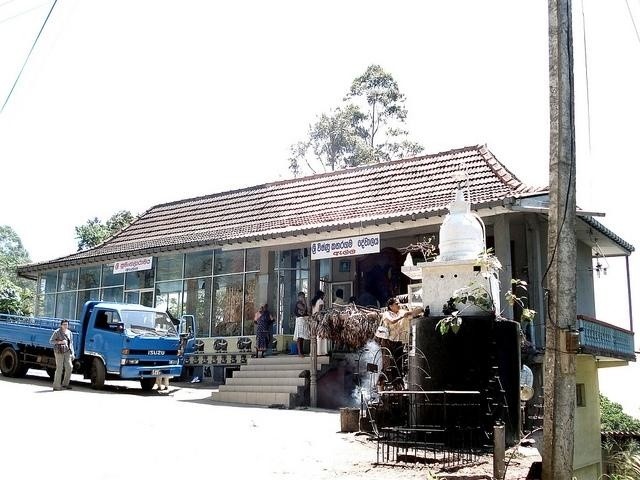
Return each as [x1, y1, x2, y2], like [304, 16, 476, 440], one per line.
[373, 390, 485, 469]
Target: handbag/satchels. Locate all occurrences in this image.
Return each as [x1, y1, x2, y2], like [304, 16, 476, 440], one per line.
[54, 344, 68, 354]
[376, 326, 389, 339]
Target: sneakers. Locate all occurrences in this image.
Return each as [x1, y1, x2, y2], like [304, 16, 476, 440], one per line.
[53, 386, 72, 390]
[157, 387, 167, 390]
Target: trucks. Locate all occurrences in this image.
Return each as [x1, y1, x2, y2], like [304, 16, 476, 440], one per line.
[0, 299, 198, 390]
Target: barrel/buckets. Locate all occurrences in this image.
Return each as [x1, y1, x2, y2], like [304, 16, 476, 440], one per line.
[339, 407, 360, 433]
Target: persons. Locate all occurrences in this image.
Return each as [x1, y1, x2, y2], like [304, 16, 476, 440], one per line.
[253, 303, 275, 357]
[521, 352, 534, 435]
[332, 288, 347, 309]
[310, 290, 330, 357]
[380, 297, 424, 392]
[348, 296, 357, 304]
[293, 291, 311, 358]
[153, 377, 170, 391]
[49, 320, 75, 391]
